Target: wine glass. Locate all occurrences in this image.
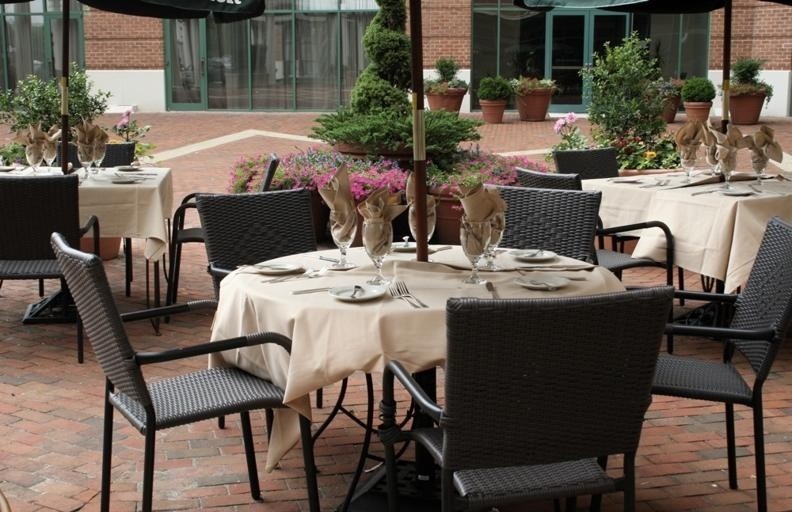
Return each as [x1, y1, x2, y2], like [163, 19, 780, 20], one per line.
[680, 144, 770, 188]
[0, 137, 157, 184]
[328, 206, 505, 285]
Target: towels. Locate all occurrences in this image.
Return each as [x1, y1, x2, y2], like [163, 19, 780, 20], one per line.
[675, 119, 783, 178]
[24, 120, 110, 165]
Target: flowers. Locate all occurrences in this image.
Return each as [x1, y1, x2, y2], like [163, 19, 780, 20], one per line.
[544, 112, 589, 162]
[110, 106, 156, 162]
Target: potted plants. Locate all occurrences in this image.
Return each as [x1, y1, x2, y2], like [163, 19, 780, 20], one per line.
[510, 75, 562, 122]
[649, 76, 683, 123]
[718, 57, 773, 126]
[305, 0, 482, 169]
[422, 56, 469, 113]
[680, 74, 716, 126]
[476, 76, 513, 123]
[224, 68, 240, 89]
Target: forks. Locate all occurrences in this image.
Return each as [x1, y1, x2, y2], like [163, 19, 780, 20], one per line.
[609, 175, 792, 196]
[530, 278, 555, 291]
[390, 280, 427, 308]
[351, 285, 361, 298]
[261, 264, 330, 283]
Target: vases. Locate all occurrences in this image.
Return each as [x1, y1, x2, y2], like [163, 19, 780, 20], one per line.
[55, 140, 136, 167]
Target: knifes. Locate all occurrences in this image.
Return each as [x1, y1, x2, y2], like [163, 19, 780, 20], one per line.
[525, 248, 541, 257]
[239, 263, 288, 270]
[485, 280, 501, 300]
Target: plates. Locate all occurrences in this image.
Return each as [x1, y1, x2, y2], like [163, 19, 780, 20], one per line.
[508, 250, 556, 262]
[514, 273, 569, 290]
[249, 261, 303, 274]
[330, 283, 387, 301]
